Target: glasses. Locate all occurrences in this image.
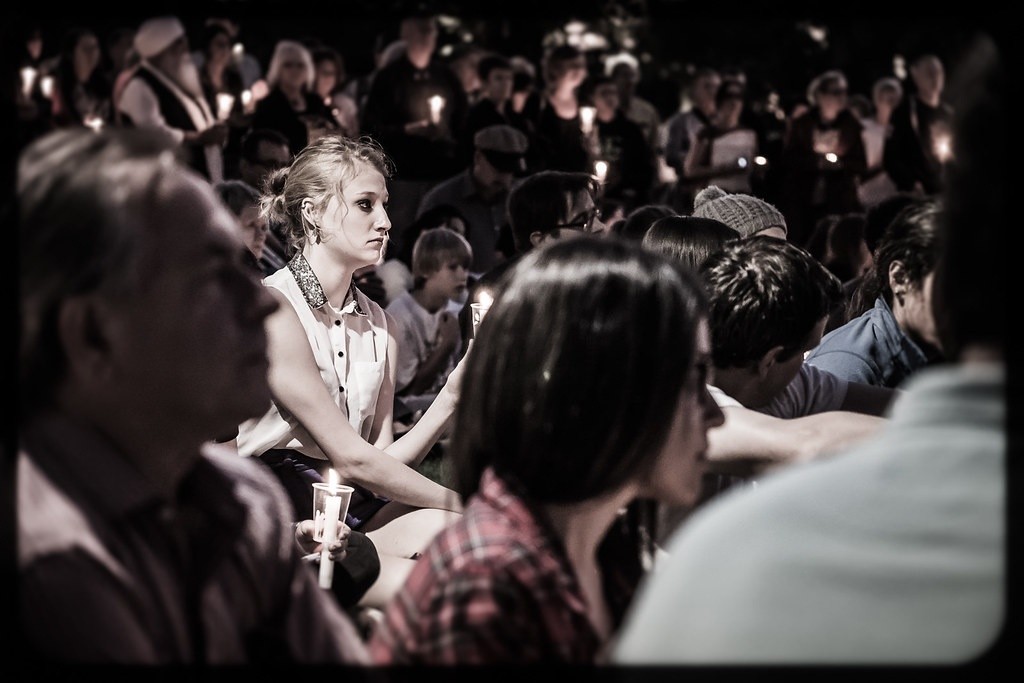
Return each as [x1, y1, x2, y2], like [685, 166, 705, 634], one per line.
[250, 158, 289, 178]
[726, 92, 742, 102]
[549, 207, 604, 233]
[281, 59, 308, 72]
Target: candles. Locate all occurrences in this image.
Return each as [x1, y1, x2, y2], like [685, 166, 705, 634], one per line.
[477, 290, 493, 322]
[317, 467, 341, 588]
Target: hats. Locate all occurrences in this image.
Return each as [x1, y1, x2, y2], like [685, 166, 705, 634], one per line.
[475, 124, 529, 172]
[690, 187, 788, 238]
[133, 16, 185, 59]
[604, 51, 640, 76]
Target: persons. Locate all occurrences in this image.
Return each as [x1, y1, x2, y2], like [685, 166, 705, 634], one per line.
[19, 0, 958, 643]
[368, 237, 727, 665]
[608, 39, 1006, 666]
[17, 130, 367, 665]
